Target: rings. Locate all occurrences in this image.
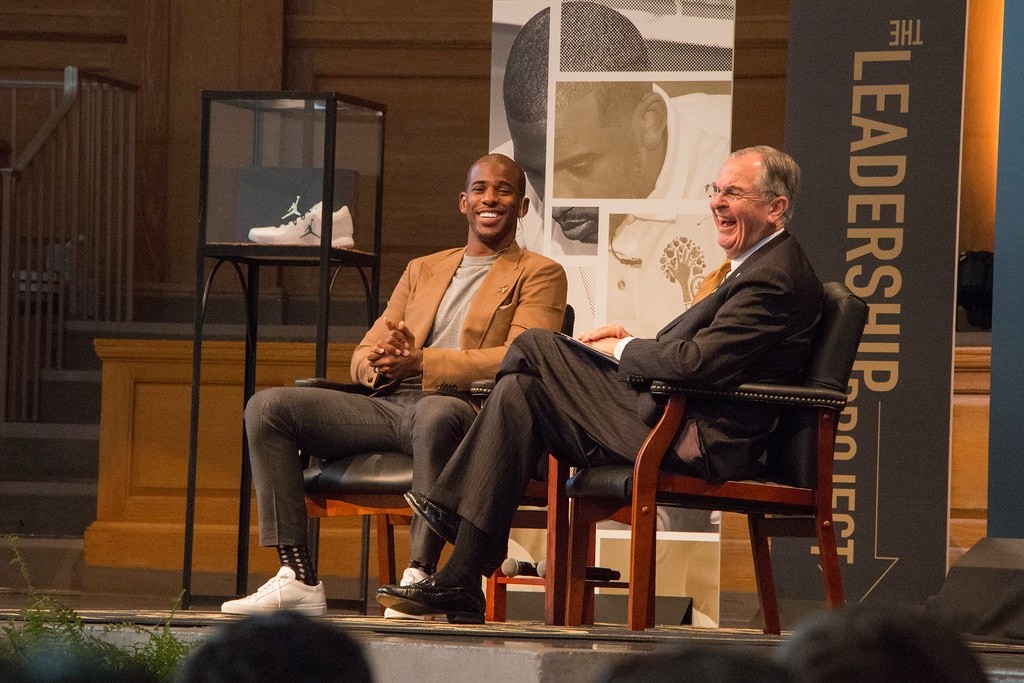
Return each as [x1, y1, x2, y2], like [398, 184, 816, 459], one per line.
[375, 367, 381, 375]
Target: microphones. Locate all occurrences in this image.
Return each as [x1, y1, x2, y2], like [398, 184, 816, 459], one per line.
[536, 559, 620, 582]
[502, 558, 540, 577]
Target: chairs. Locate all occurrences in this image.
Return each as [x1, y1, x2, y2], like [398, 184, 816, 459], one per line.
[558, 281, 870, 632]
[294, 304, 575, 621]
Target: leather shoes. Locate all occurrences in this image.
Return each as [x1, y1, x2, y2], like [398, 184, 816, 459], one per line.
[403, 488, 506, 580]
[376, 570, 486, 624]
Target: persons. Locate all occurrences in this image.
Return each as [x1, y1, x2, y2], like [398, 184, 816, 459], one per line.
[218, 151, 571, 623]
[182, 608, 375, 682]
[489, 1, 732, 363]
[375, 145, 828, 627]
[599, 597, 994, 682]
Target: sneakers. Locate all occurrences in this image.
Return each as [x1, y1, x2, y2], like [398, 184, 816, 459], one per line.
[384, 567, 431, 619]
[248, 201, 355, 249]
[221, 566, 327, 617]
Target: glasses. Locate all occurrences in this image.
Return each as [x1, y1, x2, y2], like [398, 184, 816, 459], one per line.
[705, 184, 780, 201]
